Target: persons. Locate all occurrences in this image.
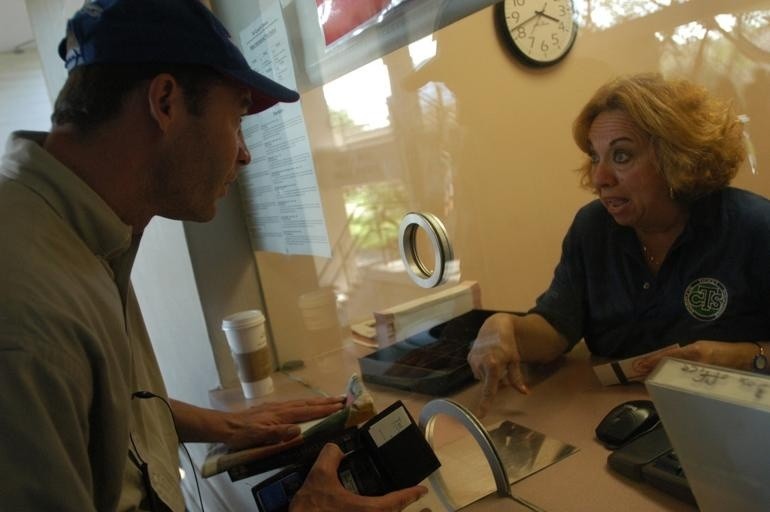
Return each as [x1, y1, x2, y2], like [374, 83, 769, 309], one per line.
[1, 1, 430, 512]
[466, 71, 768, 419]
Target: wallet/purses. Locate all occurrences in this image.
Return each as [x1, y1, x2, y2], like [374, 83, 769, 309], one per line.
[251, 400, 442, 512]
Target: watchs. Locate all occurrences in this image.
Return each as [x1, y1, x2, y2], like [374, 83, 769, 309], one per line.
[752, 340, 767, 372]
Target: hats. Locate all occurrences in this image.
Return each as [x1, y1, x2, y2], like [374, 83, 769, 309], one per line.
[55, 1, 302, 117]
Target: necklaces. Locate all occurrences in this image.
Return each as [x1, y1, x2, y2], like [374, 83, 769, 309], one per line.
[644, 246, 669, 267]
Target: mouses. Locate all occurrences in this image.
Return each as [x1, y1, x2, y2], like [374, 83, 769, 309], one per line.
[595, 399, 658, 448]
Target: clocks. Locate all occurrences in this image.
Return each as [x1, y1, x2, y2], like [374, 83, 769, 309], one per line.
[495, 0, 578, 68]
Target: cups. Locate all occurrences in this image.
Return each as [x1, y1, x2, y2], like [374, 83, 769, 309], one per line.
[221, 307, 280, 401]
